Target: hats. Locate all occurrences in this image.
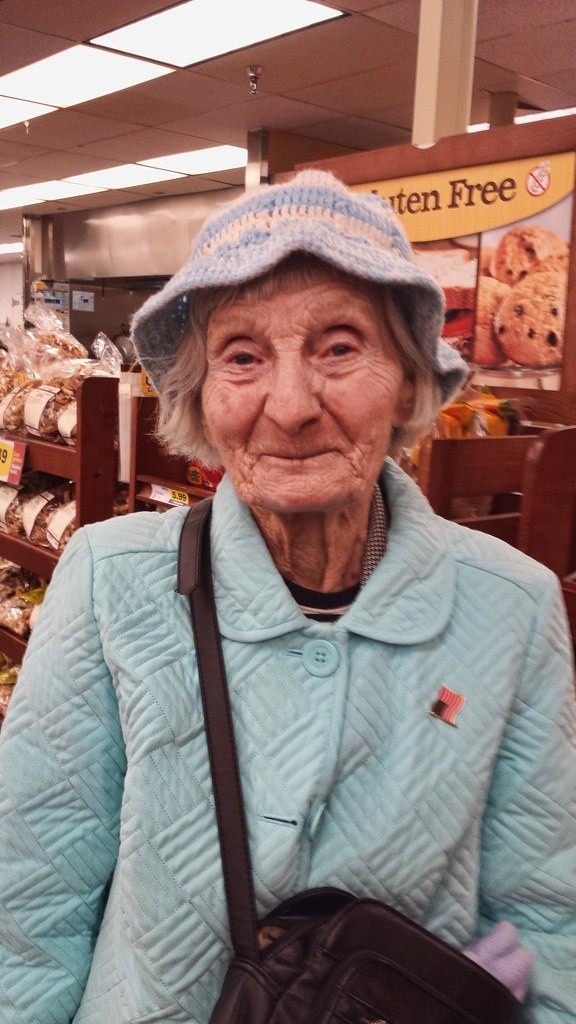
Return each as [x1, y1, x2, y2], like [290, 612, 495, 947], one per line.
[127, 169, 468, 416]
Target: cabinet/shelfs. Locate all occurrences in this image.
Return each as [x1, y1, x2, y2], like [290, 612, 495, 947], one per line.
[0, 378, 119, 664]
[127, 395, 540, 546]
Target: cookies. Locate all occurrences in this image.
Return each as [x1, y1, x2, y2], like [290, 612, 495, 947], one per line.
[472, 225, 571, 372]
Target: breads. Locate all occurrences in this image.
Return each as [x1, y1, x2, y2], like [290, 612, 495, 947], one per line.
[407, 245, 479, 312]
[0, 331, 105, 715]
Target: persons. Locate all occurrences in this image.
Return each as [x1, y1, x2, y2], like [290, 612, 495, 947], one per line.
[0, 168, 576, 1024]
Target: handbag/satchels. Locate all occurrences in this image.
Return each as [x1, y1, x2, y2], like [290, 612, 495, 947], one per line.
[205, 888, 532, 1024]
